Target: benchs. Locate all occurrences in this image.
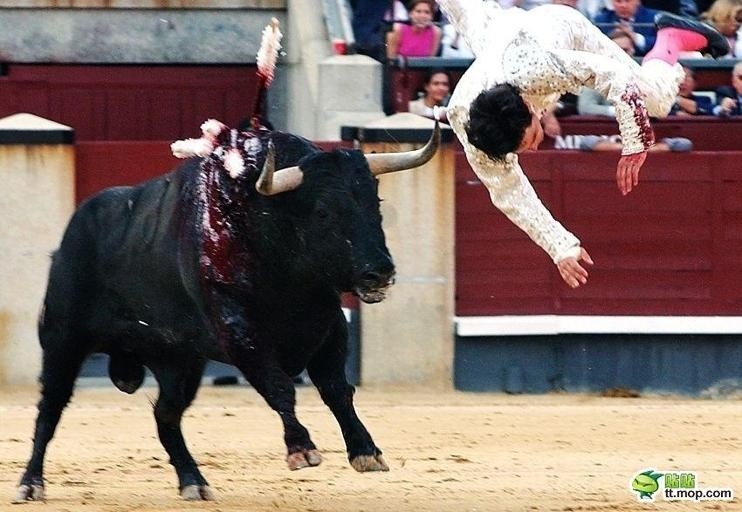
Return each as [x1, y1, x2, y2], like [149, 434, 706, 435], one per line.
[354, 1, 740, 149]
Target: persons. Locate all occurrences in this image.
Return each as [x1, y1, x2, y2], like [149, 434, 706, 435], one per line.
[497, 0, 742, 153]
[387, 2, 475, 123]
[431, 0, 733, 290]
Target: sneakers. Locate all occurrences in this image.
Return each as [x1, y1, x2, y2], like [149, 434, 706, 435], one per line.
[662, 137, 693, 151]
[653, 11, 730, 59]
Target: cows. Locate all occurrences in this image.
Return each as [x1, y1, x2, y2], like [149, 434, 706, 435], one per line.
[10, 112, 444, 504]
